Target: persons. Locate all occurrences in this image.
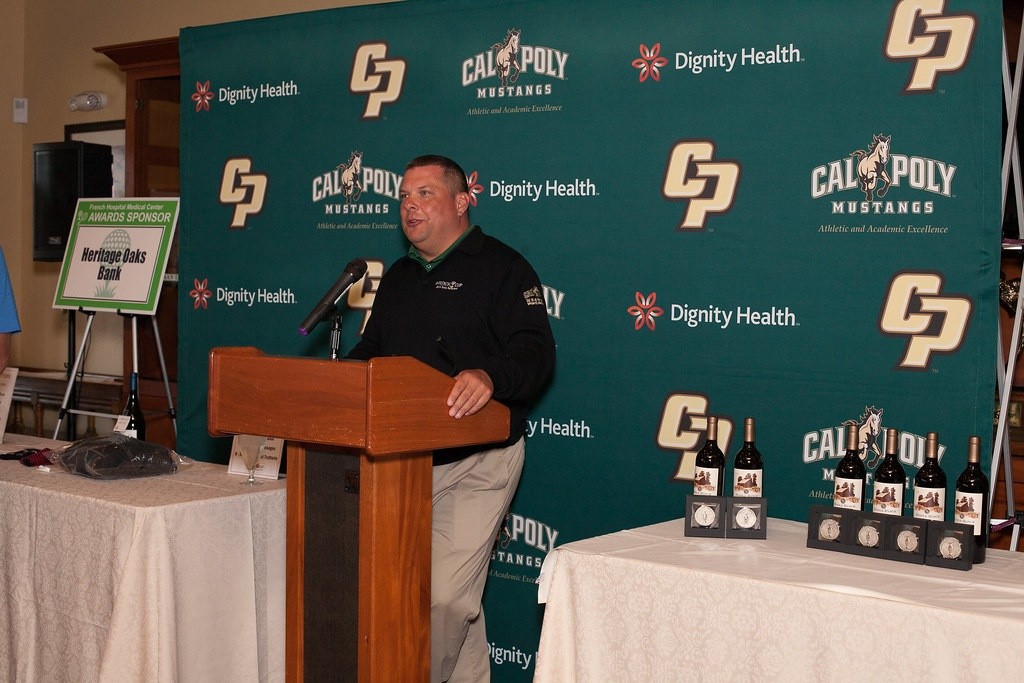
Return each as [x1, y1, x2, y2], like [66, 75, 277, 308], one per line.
[0, 245, 22, 374]
[336, 155, 556, 683]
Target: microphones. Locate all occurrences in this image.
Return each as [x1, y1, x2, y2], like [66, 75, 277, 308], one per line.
[299, 258, 368, 336]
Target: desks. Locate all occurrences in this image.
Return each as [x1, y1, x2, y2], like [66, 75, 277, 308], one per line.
[0, 433, 287, 683]
[532, 517, 1024, 683]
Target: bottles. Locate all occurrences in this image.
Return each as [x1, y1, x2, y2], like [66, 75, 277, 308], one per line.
[693, 416, 724, 496]
[914, 433, 947, 521]
[834, 425, 865, 509]
[954, 436, 988, 564]
[732, 417, 763, 496]
[873, 429, 905, 515]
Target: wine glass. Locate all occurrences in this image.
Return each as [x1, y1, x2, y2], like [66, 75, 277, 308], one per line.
[238, 436, 265, 485]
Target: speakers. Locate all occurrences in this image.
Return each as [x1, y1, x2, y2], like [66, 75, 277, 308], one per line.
[34, 141, 114, 261]
